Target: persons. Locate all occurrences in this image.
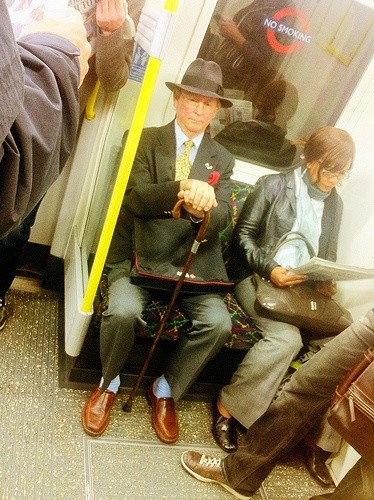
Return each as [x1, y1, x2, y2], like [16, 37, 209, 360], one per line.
[0, 0, 134, 327]
[214, 81, 298, 168]
[210, 126, 343, 485]
[83, 59, 238, 444]
[181, 308, 374, 500]
[216, 0, 296, 89]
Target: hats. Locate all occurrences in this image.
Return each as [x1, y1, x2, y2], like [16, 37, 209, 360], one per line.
[165, 58, 233, 108]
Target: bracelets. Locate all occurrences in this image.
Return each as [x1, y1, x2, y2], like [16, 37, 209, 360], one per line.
[190, 215, 203, 224]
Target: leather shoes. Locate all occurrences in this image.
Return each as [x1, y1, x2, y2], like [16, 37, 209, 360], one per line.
[305, 448, 332, 487]
[81, 384, 118, 436]
[180, 450, 256, 500]
[146, 378, 179, 444]
[213, 396, 239, 453]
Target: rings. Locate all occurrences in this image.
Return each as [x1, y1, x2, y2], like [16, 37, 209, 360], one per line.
[290, 285, 292, 287]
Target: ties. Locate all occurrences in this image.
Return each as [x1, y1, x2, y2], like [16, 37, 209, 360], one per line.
[175, 140, 194, 183]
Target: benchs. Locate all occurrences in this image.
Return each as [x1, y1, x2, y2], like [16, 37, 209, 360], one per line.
[58, 150, 306, 403]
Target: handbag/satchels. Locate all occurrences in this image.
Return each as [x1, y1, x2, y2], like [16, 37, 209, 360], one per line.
[251, 231, 354, 334]
[130, 206, 237, 295]
